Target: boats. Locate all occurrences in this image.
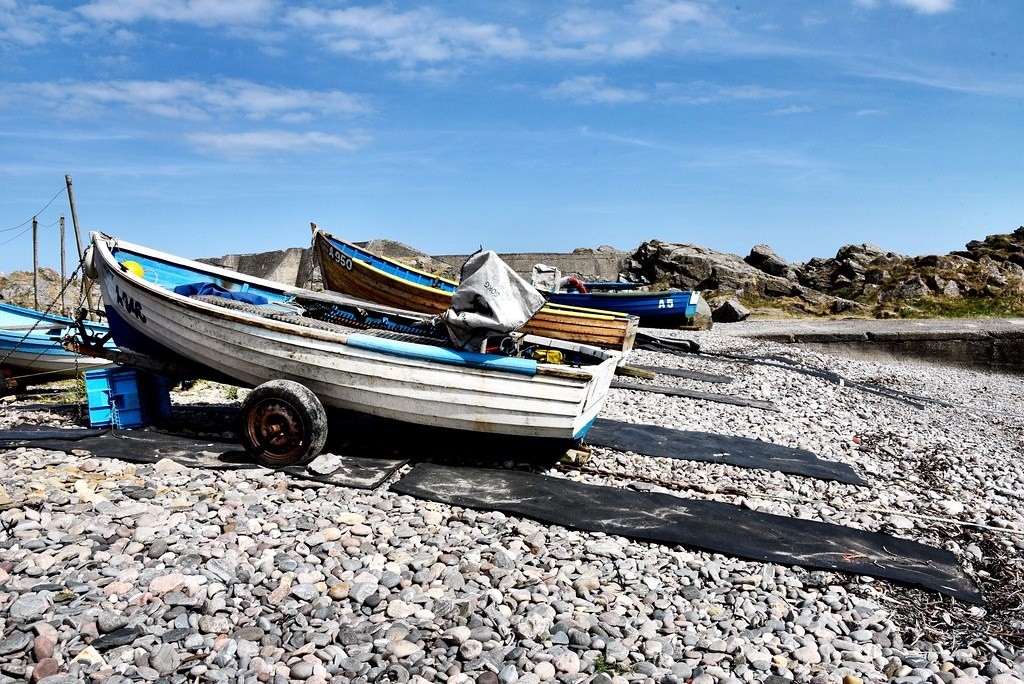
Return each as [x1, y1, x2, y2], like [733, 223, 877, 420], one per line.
[0, 302, 122, 375]
[528, 277, 702, 326]
[310, 223, 641, 356]
[87, 228, 624, 446]
